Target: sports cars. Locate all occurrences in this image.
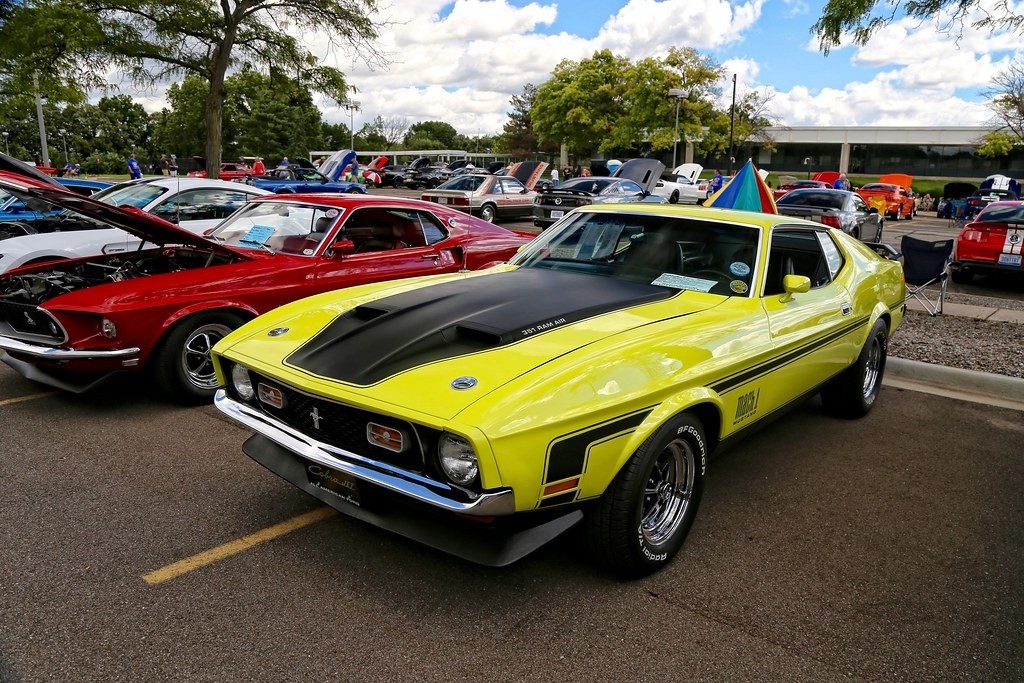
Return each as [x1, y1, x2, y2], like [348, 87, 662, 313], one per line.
[0, 169, 550, 407]
[210, 203, 907, 578]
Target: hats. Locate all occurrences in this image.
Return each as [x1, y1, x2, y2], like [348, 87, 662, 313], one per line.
[171, 154, 176, 159]
[284, 157, 288, 160]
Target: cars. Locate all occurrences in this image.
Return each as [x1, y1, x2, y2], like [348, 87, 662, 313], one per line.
[187, 149, 553, 194]
[0, 152, 329, 273]
[420, 161, 550, 223]
[773, 171, 917, 221]
[0, 178, 175, 222]
[590, 158, 736, 205]
[951, 200, 1024, 285]
[532, 158, 670, 245]
[937, 174, 1023, 220]
[775, 188, 884, 253]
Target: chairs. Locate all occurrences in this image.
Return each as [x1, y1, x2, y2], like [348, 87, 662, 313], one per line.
[355, 221, 404, 254]
[307, 217, 346, 244]
[625, 232, 676, 275]
[885, 235, 957, 317]
[728, 248, 795, 295]
[948, 202, 967, 228]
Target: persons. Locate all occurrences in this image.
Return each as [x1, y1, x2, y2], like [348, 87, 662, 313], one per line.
[834, 174, 853, 191]
[128, 154, 140, 180]
[914, 193, 933, 211]
[319, 157, 325, 167]
[551, 163, 590, 186]
[239, 155, 291, 175]
[160, 156, 178, 176]
[708, 169, 723, 193]
[348, 157, 358, 183]
[506, 162, 514, 169]
[466, 162, 474, 167]
[35, 155, 80, 176]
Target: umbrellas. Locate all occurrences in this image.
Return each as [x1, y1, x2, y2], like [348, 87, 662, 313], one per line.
[703, 158, 779, 213]
[363, 171, 382, 183]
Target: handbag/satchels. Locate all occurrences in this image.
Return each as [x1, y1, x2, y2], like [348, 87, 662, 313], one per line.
[139, 172, 143, 178]
